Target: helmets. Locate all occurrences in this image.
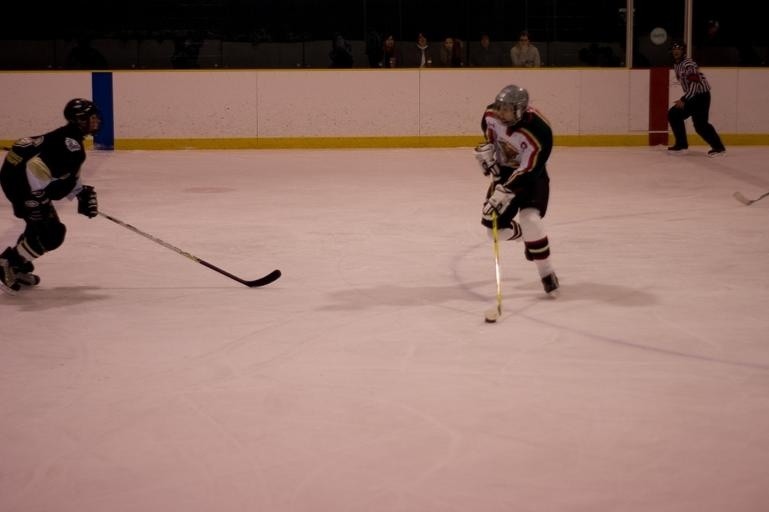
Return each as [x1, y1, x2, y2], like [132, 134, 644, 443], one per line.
[65, 100, 97, 120]
[496, 85, 528, 120]
[672, 42, 686, 51]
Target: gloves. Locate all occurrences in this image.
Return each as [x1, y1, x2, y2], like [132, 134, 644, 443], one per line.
[483, 184, 515, 221]
[473, 143, 500, 178]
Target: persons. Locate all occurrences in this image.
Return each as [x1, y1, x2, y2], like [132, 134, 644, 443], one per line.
[406, 31, 434, 69]
[329, 32, 353, 68]
[664, 40, 726, 155]
[477, 32, 502, 67]
[0, 95, 101, 291]
[369, 34, 402, 69]
[509, 30, 541, 68]
[439, 36, 466, 67]
[474, 84, 563, 301]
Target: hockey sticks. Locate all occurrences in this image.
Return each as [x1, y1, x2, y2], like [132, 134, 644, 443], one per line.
[486, 171, 503, 323]
[733, 193, 769, 206]
[98, 210, 282, 287]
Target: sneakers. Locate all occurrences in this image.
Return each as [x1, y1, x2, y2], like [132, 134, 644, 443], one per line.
[708, 147, 725, 154]
[542, 272, 559, 292]
[668, 144, 688, 151]
[0, 247, 39, 291]
